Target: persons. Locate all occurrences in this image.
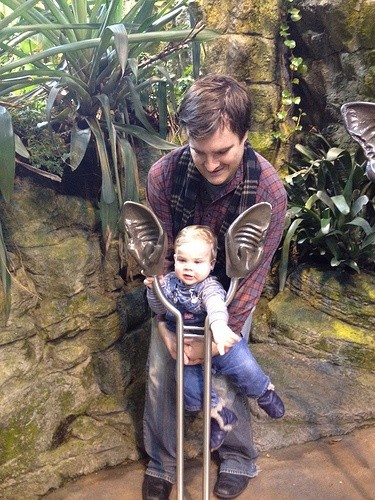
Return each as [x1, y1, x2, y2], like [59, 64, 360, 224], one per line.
[141, 73, 288, 500]
[141, 225, 286, 451]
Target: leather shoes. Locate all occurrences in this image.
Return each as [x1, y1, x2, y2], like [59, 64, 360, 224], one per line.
[214, 472, 249, 498]
[142, 474, 173, 500]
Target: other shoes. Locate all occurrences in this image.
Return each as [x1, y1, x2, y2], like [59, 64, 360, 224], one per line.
[257, 384, 285, 419]
[210, 403, 238, 453]
